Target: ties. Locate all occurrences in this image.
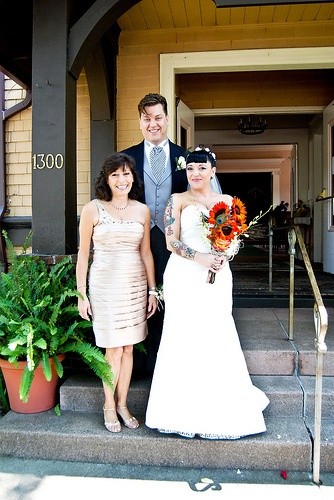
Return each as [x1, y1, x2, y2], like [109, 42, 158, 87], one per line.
[149, 147, 165, 183]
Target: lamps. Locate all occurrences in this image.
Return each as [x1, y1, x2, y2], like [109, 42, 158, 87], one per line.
[236, 113, 268, 135]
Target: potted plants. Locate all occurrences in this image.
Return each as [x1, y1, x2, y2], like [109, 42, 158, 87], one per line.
[0, 226, 148, 414]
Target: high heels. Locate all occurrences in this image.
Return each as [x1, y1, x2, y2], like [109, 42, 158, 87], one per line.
[101, 404, 122, 433]
[116, 406, 139, 429]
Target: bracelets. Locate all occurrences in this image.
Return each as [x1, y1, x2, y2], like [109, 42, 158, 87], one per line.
[148, 288, 160, 296]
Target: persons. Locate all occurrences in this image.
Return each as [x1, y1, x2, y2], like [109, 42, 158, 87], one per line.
[119, 93, 192, 361]
[76, 153, 159, 432]
[145, 147, 270, 440]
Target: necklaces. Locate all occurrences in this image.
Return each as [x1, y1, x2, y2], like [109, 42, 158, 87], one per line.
[109, 200, 129, 210]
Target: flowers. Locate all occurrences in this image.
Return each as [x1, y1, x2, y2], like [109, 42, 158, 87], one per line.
[201, 201, 275, 283]
